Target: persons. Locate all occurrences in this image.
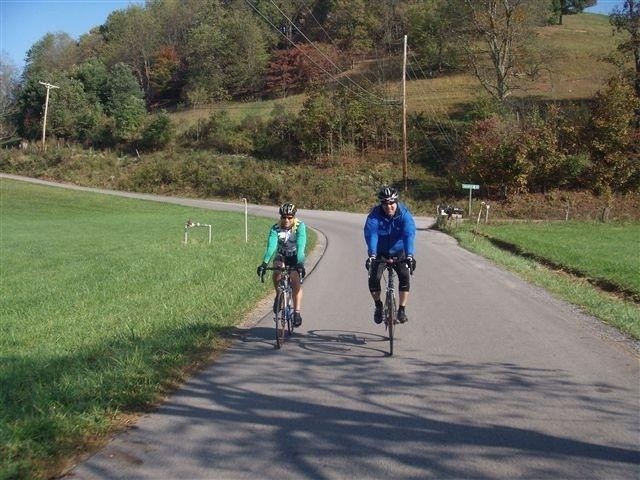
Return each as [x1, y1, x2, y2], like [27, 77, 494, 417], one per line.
[362, 187, 417, 325]
[255, 202, 308, 328]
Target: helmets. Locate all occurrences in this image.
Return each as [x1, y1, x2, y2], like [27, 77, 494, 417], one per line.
[279, 203, 298, 216]
[377, 186, 399, 202]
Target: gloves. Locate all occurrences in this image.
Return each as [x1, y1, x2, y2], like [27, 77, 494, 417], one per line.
[257, 261, 268, 276]
[366, 255, 376, 271]
[405, 255, 416, 271]
[296, 262, 305, 278]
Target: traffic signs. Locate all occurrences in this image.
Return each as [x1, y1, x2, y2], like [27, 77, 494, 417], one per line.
[461, 184, 480, 190]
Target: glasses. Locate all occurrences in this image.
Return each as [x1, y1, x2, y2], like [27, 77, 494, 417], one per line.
[282, 216, 293, 220]
[383, 200, 396, 206]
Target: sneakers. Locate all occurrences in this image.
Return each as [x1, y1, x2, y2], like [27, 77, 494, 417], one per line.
[273, 293, 284, 313]
[374, 302, 384, 324]
[397, 309, 407, 323]
[292, 313, 302, 326]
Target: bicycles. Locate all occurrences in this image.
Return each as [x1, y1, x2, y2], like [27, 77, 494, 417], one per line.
[366, 257, 415, 357]
[258, 266, 304, 350]
